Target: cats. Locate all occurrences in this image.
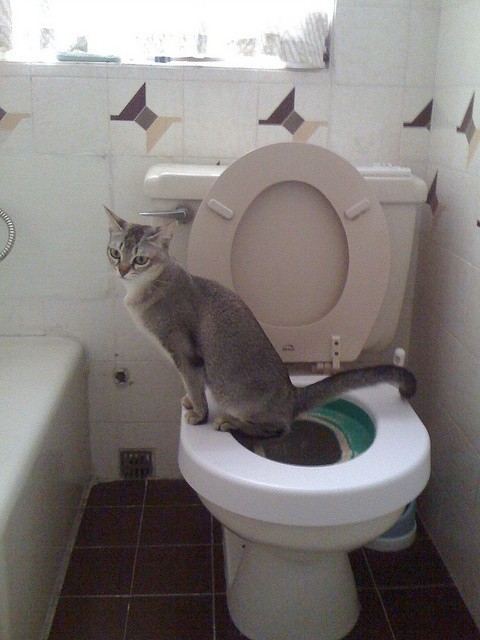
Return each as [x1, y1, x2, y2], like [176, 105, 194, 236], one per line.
[102, 203, 416, 441]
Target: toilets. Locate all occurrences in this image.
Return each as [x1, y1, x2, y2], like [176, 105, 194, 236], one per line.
[144, 162, 434, 637]
